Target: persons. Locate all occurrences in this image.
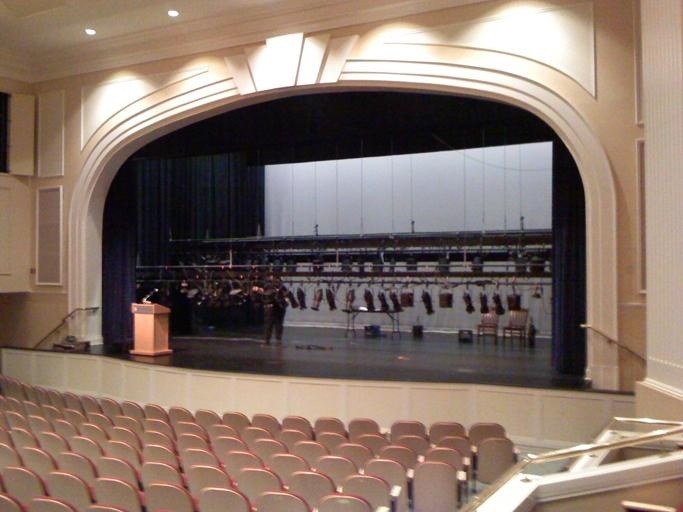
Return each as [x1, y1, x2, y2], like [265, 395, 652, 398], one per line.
[264, 276, 289, 346]
[247, 257, 264, 328]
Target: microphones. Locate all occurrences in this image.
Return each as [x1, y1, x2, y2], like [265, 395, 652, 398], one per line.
[150, 289, 158, 296]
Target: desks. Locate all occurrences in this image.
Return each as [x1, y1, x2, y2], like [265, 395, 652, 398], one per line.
[341, 305, 403, 341]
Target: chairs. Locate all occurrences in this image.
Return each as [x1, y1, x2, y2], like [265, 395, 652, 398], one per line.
[0, 373, 518, 512]
[502, 308, 528, 348]
[477, 308, 498, 345]
